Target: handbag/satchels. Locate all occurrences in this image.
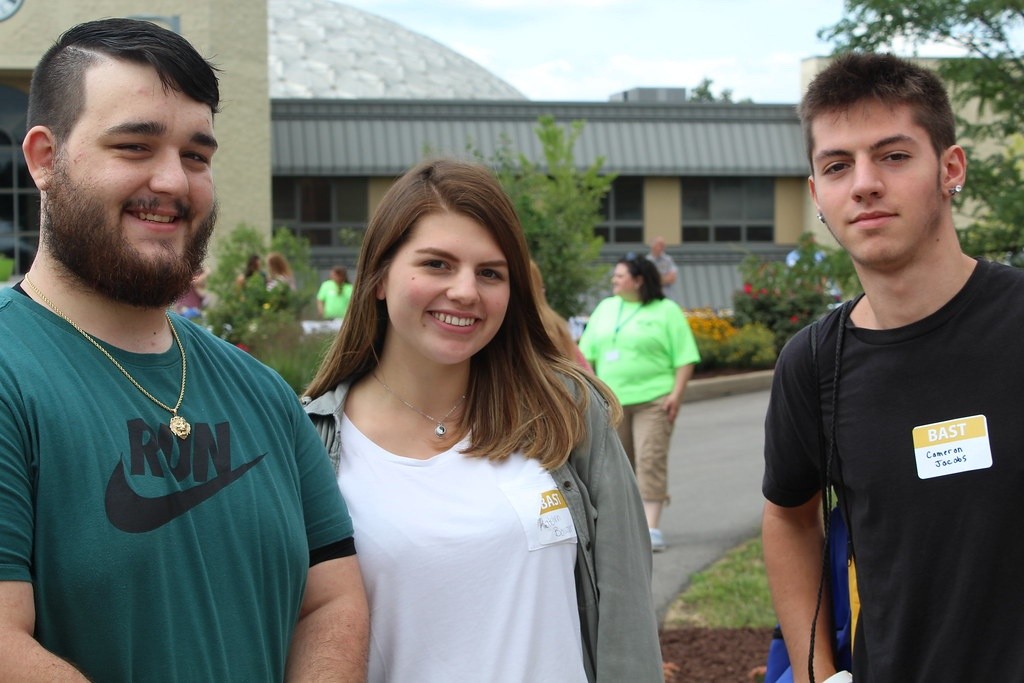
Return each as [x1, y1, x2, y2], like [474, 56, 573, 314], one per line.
[764, 504, 860, 683]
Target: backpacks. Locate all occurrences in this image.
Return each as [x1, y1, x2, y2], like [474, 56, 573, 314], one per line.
[271, 276, 290, 312]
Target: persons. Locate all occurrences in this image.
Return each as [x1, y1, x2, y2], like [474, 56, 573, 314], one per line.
[645, 237, 680, 301]
[0, 18, 366, 683]
[578, 252, 700, 552]
[316, 266, 354, 322]
[761, 52, 1024, 683]
[178, 261, 210, 318]
[787, 231, 839, 295]
[302, 160, 666, 683]
[236, 254, 295, 293]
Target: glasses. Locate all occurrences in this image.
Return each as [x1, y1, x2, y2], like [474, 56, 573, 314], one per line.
[621, 251, 640, 270]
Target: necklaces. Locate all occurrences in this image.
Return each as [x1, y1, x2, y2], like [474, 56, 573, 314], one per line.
[373, 374, 467, 437]
[23, 272, 192, 440]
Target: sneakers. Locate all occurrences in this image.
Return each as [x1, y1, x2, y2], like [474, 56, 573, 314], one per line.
[649, 528, 665, 550]
